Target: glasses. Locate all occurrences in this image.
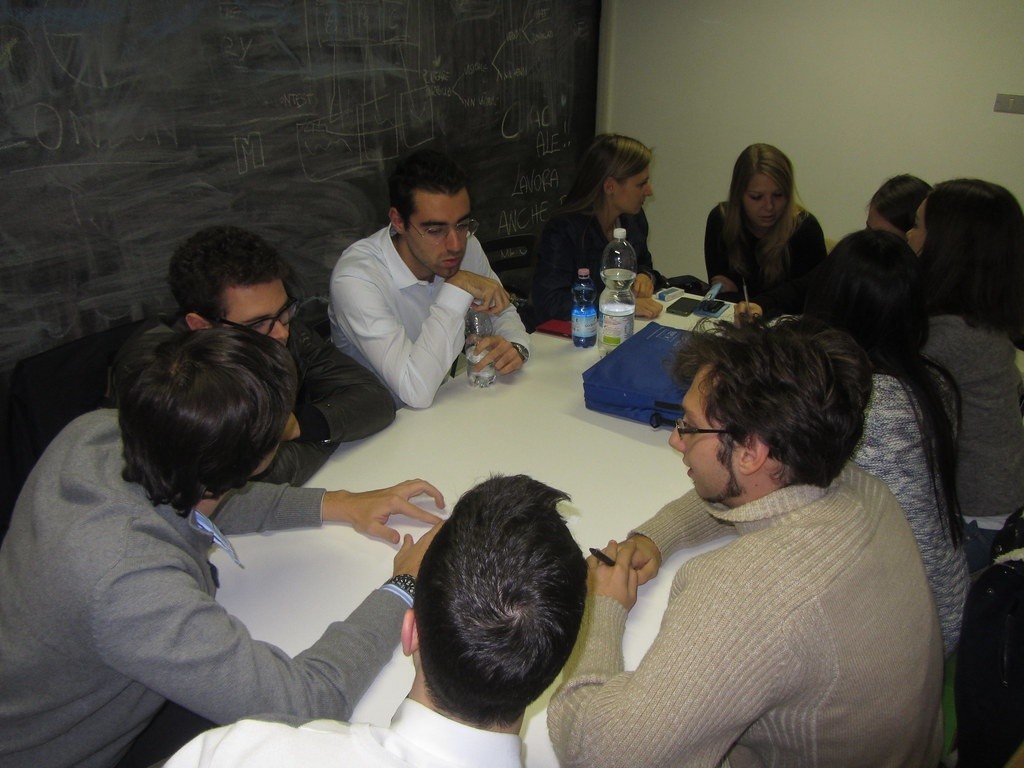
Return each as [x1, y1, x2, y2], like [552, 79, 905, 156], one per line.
[216, 297, 301, 336]
[675, 419, 727, 440]
[408, 219, 479, 245]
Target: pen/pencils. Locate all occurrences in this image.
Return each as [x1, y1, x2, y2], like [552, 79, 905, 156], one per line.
[742, 277, 750, 311]
[589, 548, 615, 566]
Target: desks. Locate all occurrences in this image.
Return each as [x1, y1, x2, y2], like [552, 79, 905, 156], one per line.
[209, 288, 736, 768]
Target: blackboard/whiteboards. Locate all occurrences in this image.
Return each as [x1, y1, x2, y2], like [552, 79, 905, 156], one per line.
[0, 0, 604, 539]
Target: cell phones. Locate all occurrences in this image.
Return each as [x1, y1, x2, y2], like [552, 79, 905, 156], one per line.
[666, 297, 701, 317]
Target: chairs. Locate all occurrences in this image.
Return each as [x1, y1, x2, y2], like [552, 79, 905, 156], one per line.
[479, 236, 534, 300]
[13, 318, 157, 484]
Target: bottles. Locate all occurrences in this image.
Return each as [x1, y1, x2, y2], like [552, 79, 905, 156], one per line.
[464, 299, 497, 387]
[571, 268, 597, 348]
[599, 227, 635, 360]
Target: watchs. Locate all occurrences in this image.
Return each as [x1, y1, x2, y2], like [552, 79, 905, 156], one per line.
[512, 342, 529, 363]
[383, 574, 416, 599]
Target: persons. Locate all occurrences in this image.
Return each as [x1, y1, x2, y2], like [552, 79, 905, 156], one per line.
[704, 143, 828, 329]
[803, 174, 1024, 663]
[0, 323, 445, 768]
[547, 313, 944, 768]
[160, 473, 589, 767]
[94, 225, 396, 488]
[326, 148, 531, 409]
[536, 134, 666, 321]
